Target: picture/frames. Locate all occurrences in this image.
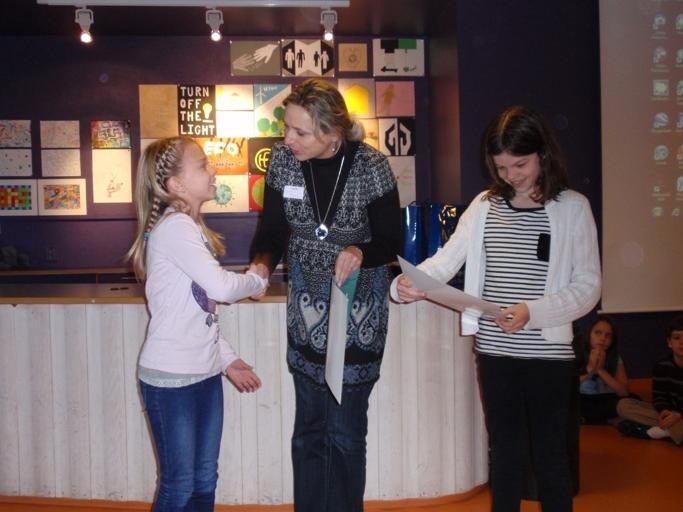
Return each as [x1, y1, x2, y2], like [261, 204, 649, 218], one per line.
[0, 179, 38, 216]
[37, 179, 87, 216]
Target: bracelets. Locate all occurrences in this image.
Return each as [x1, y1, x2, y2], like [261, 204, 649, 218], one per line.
[348, 245, 363, 255]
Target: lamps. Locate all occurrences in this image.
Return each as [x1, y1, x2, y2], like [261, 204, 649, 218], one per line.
[36, 0, 349, 44]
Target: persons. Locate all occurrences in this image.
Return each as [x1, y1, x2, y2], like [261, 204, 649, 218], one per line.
[388, 106, 601, 509]
[616, 323, 682, 446]
[578, 317, 641, 419]
[123, 134, 270, 510]
[246, 83, 403, 510]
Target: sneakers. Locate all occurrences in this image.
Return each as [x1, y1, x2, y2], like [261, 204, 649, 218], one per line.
[617, 420, 654, 440]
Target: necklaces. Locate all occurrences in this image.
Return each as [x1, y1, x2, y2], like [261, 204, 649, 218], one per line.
[307, 159, 343, 241]
